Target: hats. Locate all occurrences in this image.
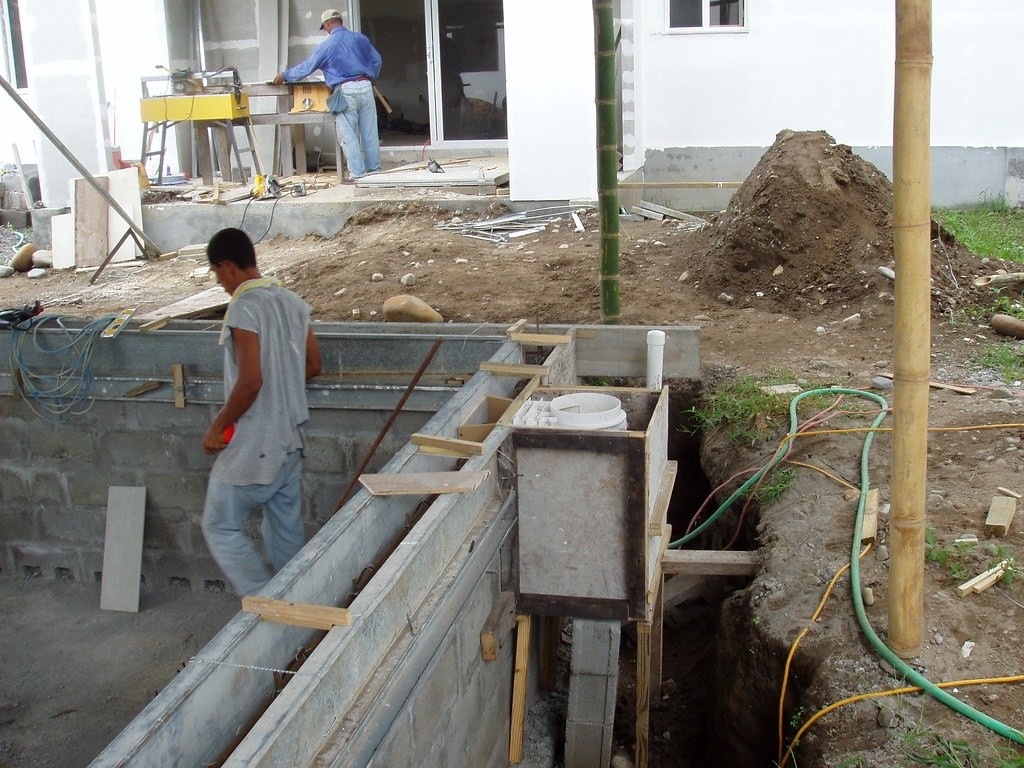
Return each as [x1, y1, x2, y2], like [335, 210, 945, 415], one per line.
[318, 8, 343, 31]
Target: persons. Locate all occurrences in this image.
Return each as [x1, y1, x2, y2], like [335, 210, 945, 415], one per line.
[200, 227, 321, 601]
[274, 9, 382, 181]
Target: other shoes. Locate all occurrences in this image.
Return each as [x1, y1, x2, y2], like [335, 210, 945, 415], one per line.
[340, 177, 359, 185]
